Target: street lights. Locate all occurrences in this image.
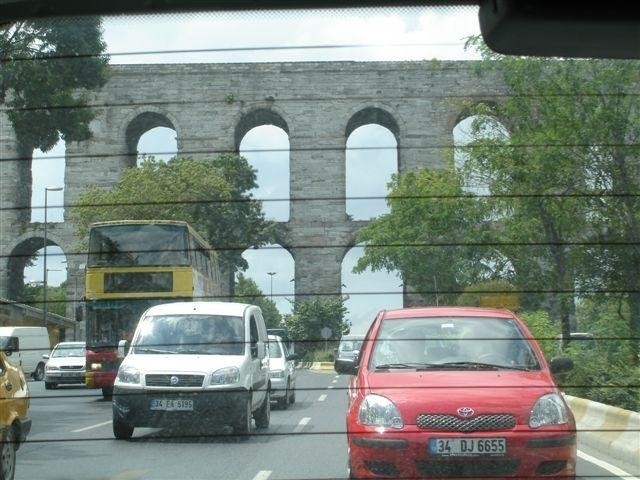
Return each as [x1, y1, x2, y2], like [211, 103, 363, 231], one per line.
[43, 183, 65, 326]
[266, 271, 278, 302]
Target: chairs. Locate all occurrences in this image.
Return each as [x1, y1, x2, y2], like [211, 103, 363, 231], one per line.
[382, 329, 431, 363]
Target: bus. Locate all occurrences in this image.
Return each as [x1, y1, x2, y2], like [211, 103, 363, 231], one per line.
[82, 218, 225, 399]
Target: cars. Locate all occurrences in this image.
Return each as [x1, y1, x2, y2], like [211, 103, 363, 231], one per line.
[40, 340, 88, 391]
[266, 333, 299, 411]
[332, 304, 583, 479]
[332, 333, 366, 374]
[0, 349, 33, 480]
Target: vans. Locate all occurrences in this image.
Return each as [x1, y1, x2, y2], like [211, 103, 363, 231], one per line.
[0, 326, 52, 383]
[106, 300, 274, 439]
